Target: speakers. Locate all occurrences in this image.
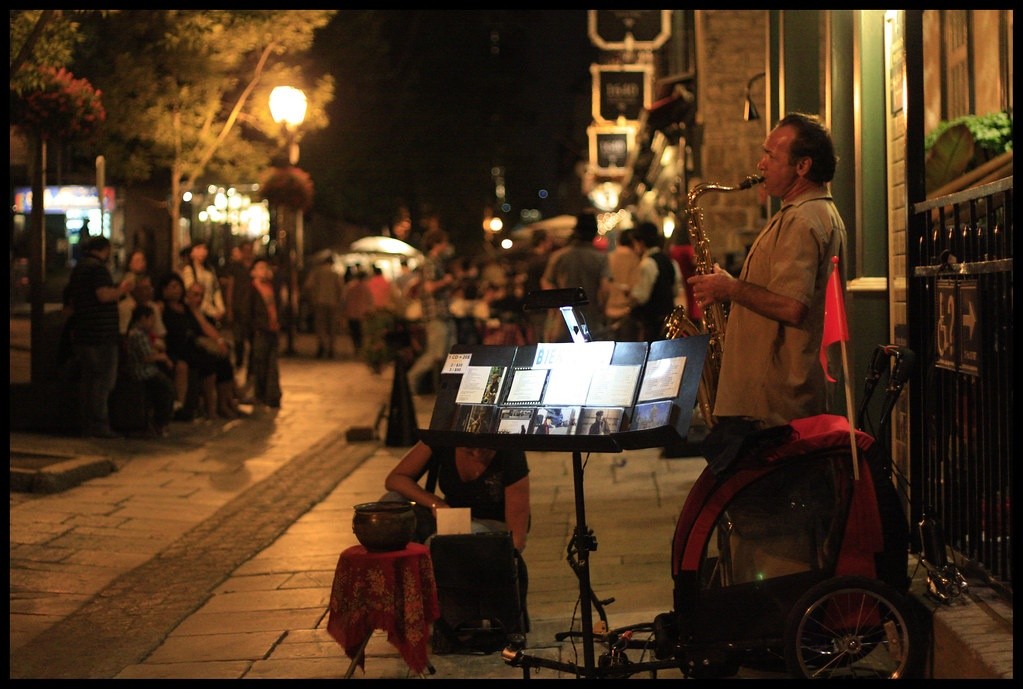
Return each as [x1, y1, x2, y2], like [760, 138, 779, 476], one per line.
[429, 530, 527, 655]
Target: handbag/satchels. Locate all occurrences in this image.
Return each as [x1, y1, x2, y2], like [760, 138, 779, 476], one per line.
[407, 448, 438, 543]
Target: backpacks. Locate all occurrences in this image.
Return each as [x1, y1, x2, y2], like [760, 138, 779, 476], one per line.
[431, 532, 529, 655]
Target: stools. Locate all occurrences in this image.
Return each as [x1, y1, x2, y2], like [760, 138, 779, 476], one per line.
[331, 541, 438, 677]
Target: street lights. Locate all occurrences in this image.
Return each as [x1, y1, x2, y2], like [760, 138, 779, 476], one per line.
[270, 82, 309, 355]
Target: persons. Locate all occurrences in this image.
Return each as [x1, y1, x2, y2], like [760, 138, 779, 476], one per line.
[385, 438, 531, 644]
[69, 236, 136, 438]
[302, 207, 686, 400]
[112, 239, 282, 433]
[688, 111, 848, 429]
[534, 410, 610, 435]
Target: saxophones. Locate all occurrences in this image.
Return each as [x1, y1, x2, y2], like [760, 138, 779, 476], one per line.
[659, 171, 766, 431]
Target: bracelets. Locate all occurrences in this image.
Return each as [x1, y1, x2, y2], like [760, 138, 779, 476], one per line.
[432, 495, 440, 519]
[216, 337, 224, 344]
